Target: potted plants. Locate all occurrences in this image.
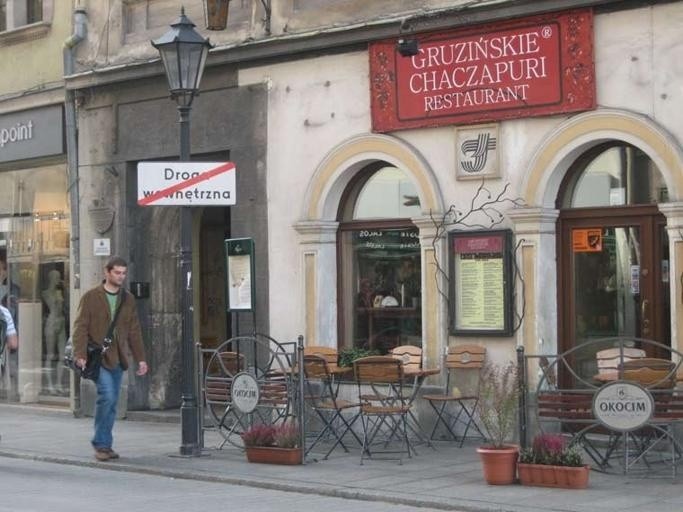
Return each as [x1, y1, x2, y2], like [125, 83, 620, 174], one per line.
[469, 362, 528, 486]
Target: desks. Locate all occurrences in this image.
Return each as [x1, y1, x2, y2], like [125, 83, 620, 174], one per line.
[356, 306, 420, 349]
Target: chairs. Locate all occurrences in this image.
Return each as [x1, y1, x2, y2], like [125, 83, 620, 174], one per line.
[537, 344, 682, 482]
[205, 343, 489, 466]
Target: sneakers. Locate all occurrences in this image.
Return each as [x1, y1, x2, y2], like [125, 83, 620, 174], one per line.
[96, 448, 118, 461]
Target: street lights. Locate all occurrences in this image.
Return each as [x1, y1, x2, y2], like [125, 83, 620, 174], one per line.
[145, 5, 218, 459]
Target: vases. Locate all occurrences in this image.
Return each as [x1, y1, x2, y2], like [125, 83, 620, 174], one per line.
[515, 462, 589, 488]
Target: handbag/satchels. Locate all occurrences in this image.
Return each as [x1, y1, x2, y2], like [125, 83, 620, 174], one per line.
[65, 333, 103, 381]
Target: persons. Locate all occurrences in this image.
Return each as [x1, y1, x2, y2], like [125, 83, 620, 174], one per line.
[42, 269, 67, 395]
[353, 278, 371, 350]
[0, 305, 18, 441]
[70, 255, 149, 460]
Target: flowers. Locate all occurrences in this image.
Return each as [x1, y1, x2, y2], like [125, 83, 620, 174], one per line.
[531, 432, 567, 457]
